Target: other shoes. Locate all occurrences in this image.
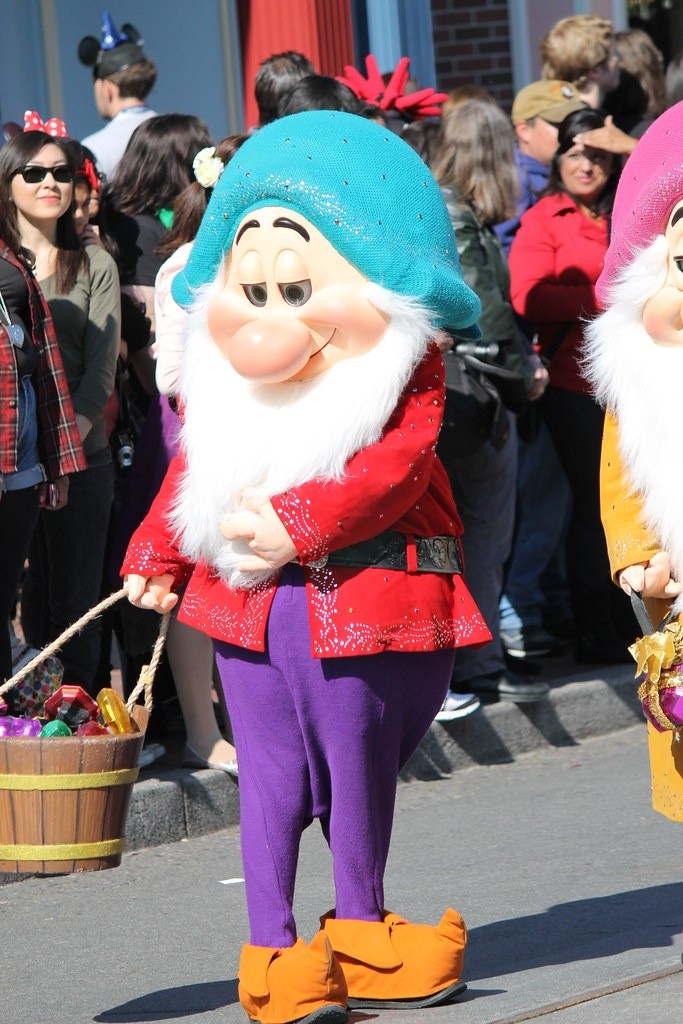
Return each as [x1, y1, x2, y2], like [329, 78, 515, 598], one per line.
[139, 743, 167, 770]
[182, 740, 240, 778]
[432, 689, 480, 721]
[453, 667, 551, 702]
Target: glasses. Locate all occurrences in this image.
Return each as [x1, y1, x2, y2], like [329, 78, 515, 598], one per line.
[15, 165, 73, 183]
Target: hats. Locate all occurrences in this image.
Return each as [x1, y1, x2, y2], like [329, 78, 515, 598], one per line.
[78, 12, 148, 81]
[510, 80, 594, 125]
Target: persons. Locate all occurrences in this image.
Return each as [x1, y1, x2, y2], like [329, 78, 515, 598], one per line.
[275, 72, 361, 123]
[96, 116, 213, 750]
[254, 52, 313, 124]
[114, 111, 477, 1020]
[84, 43, 164, 182]
[427, 89, 550, 721]
[543, 15, 651, 136]
[492, 80, 588, 257]
[501, 111, 641, 671]
[0, 179, 87, 716]
[1, 127, 122, 701]
[583, 100, 683, 824]
[608, 27, 664, 119]
